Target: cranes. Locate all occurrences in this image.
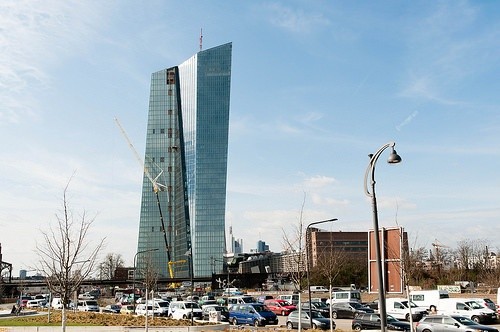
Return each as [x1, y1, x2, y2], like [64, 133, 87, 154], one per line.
[432, 238, 451, 259]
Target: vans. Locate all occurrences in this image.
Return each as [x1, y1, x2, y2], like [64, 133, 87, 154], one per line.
[168, 301, 203, 319]
[405, 290, 449, 311]
[228, 303, 278, 327]
[158, 284, 379, 316]
[378, 298, 428, 322]
[436, 298, 497, 324]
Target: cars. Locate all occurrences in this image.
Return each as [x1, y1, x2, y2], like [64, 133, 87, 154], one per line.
[202, 306, 229, 321]
[416, 314, 499, 332]
[286, 310, 336, 330]
[20, 294, 169, 317]
[352, 313, 410, 332]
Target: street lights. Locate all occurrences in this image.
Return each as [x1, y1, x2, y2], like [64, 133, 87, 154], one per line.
[363, 141, 402, 332]
[134, 249, 157, 316]
[306, 218, 338, 332]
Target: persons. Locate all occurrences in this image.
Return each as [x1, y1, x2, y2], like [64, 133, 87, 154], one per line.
[11, 305, 22, 315]
[177, 295, 183, 301]
